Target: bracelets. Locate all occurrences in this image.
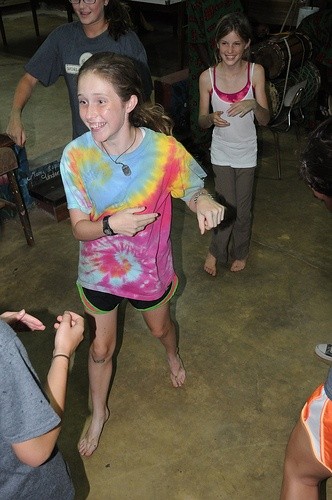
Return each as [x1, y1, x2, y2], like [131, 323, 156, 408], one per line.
[208, 113, 212, 125]
[193, 191, 213, 205]
[52, 354, 70, 360]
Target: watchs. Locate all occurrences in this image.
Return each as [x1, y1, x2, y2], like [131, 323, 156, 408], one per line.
[102, 214, 114, 236]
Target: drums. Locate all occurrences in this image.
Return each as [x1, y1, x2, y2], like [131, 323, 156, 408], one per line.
[257, 60, 320, 128]
[252, 32, 313, 81]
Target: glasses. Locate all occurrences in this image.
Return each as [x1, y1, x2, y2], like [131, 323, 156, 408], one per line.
[69, 0, 96, 4]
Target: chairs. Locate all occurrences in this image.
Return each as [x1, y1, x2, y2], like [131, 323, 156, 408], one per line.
[256, 87, 304, 181]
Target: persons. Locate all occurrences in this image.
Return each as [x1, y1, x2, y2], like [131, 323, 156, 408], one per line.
[197, 16, 269, 275]
[311, 187, 332, 362]
[283, 366, 331, 499]
[0, 308, 84, 500]
[60, 53, 224, 458]
[6, 0, 148, 149]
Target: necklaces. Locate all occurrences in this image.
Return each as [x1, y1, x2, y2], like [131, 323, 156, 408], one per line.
[100, 127, 137, 175]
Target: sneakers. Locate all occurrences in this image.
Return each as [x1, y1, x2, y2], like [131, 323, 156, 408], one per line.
[314, 343, 332, 362]
